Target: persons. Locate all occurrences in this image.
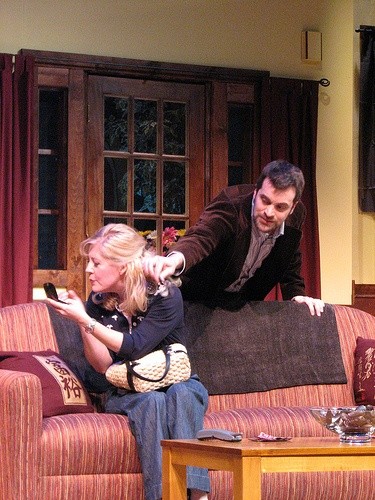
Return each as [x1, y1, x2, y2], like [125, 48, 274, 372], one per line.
[43, 222, 210, 500]
[145, 160, 328, 317]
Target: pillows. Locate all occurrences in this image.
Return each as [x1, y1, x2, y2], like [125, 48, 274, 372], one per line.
[353, 337, 374, 407]
[0, 349, 96, 417]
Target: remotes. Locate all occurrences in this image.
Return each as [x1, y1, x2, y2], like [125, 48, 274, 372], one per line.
[197, 430, 243, 442]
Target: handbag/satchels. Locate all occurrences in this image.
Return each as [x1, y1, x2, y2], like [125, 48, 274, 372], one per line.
[104, 343, 191, 390]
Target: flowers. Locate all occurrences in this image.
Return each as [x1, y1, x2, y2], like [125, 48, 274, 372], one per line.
[138, 227, 186, 255]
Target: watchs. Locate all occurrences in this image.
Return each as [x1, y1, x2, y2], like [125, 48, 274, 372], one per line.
[84, 320, 96, 334]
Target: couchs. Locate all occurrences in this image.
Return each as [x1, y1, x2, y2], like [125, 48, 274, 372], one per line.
[0, 300, 375, 500]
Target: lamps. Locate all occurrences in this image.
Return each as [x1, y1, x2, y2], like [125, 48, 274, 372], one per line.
[302, 30, 324, 62]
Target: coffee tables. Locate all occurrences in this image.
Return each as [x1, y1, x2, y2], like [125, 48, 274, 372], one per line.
[160, 439, 375, 500]
